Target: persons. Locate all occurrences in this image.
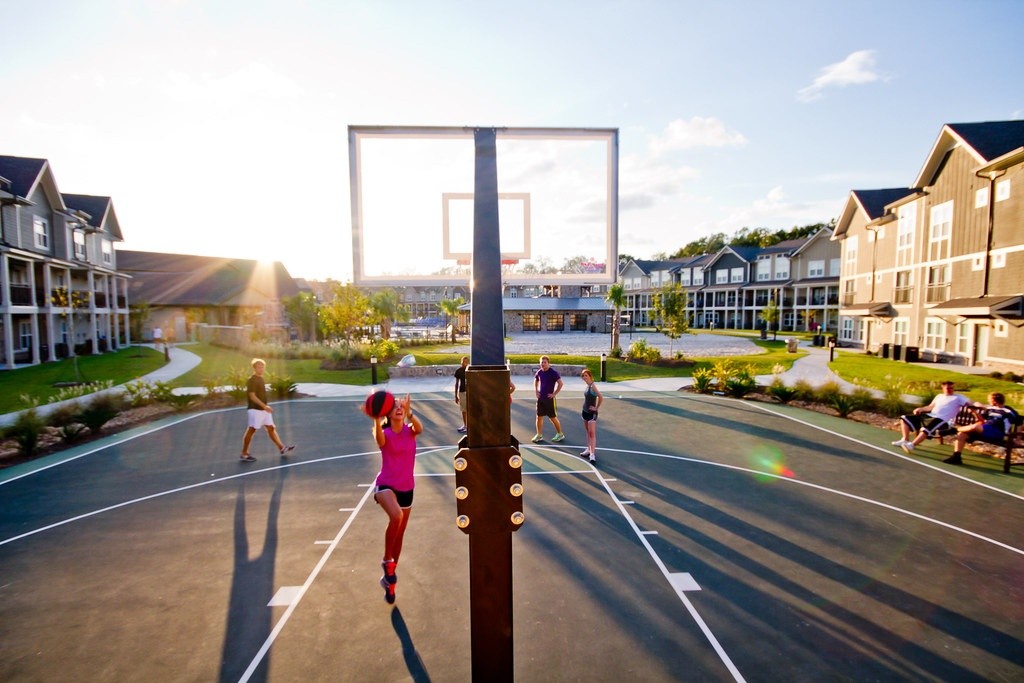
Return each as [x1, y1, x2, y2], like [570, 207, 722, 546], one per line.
[239, 359, 295, 461]
[942, 393, 1023, 465]
[371, 393, 423, 605]
[510, 379, 515, 416]
[579, 369, 603, 462]
[531, 355, 565, 442]
[152, 325, 174, 348]
[454, 357, 468, 432]
[891, 381, 973, 454]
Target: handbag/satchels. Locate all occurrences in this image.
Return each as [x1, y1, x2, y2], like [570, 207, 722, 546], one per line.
[397, 354, 416, 368]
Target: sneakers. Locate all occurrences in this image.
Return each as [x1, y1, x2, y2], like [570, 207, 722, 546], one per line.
[552, 432, 566, 442]
[531, 434, 543, 443]
[381, 558, 397, 585]
[380, 576, 395, 604]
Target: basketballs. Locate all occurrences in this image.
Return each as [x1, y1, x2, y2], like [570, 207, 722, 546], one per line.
[365, 391, 394, 418]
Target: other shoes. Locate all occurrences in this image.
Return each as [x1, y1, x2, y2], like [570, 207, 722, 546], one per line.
[892, 438, 909, 446]
[942, 451, 963, 465]
[938, 428, 958, 436]
[589, 453, 596, 461]
[239, 455, 257, 461]
[281, 445, 295, 455]
[579, 449, 590, 457]
[901, 443, 914, 454]
[458, 425, 467, 433]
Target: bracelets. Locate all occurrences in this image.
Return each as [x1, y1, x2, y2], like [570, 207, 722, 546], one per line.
[407, 410, 412, 419]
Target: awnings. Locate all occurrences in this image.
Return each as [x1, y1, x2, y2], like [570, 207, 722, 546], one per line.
[928, 296, 1022, 328]
[839, 302, 890, 321]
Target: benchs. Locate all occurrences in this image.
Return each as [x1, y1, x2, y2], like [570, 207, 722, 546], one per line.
[937, 405, 1023, 474]
